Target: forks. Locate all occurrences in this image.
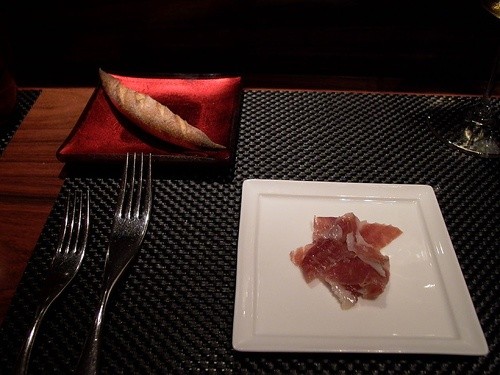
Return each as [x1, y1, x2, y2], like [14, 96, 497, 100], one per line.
[75, 152, 152, 375]
[13, 190, 90, 374]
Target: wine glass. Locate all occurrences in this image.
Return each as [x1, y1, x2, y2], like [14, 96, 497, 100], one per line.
[428, 0, 500, 156]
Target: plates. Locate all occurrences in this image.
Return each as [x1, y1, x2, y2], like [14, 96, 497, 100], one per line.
[56, 72, 242, 165]
[232, 179, 489, 356]
